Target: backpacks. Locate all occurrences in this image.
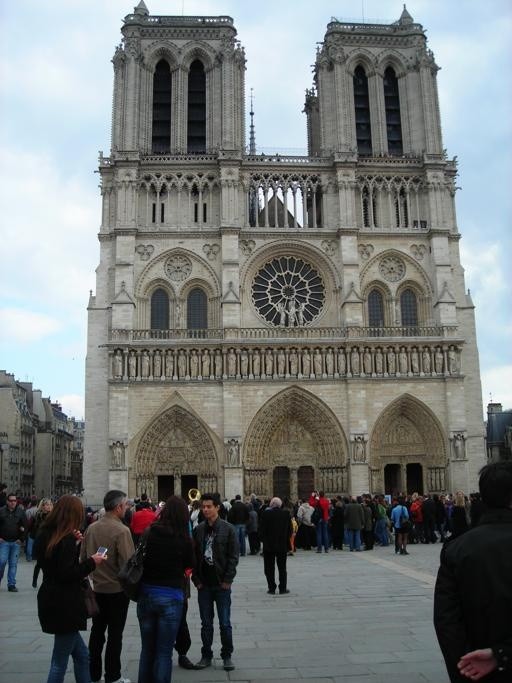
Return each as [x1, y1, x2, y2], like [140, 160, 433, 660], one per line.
[311, 501, 324, 526]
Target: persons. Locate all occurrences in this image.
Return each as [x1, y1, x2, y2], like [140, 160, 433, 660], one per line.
[275, 300, 306, 327]
[79, 489, 139, 683]
[199, 476, 218, 493]
[1, 482, 105, 592]
[174, 578, 196, 670]
[242, 469, 268, 496]
[124, 489, 481, 556]
[135, 494, 193, 683]
[227, 439, 241, 466]
[194, 492, 242, 671]
[31, 493, 110, 683]
[453, 434, 466, 460]
[111, 438, 123, 468]
[111, 343, 459, 381]
[354, 438, 366, 462]
[320, 465, 348, 492]
[432, 460, 512, 683]
[426, 467, 447, 490]
[134, 477, 156, 499]
[257, 496, 294, 595]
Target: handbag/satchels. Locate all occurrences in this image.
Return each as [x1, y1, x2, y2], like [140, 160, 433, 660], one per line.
[119, 546, 145, 602]
[400, 511, 410, 533]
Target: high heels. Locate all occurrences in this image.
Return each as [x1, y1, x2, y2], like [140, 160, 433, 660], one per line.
[402, 549, 410, 555]
[395, 549, 401, 554]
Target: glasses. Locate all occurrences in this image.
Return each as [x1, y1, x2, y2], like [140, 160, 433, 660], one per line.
[8, 498, 17, 502]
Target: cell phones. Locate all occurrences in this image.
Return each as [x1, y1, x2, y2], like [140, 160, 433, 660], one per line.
[96, 547, 108, 556]
[314, 490, 317, 493]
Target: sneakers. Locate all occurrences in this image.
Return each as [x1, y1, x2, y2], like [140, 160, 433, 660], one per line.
[32, 581, 37, 587]
[279, 589, 289, 593]
[240, 554, 245, 556]
[288, 552, 293, 556]
[325, 549, 329, 552]
[267, 590, 275, 594]
[8, 585, 18, 592]
[195, 657, 211, 669]
[260, 553, 264, 556]
[223, 660, 235, 671]
[248, 552, 257, 555]
[114, 677, 130, 683]
[316, 550, 322, 553]
[178, 657, 194, 670]
